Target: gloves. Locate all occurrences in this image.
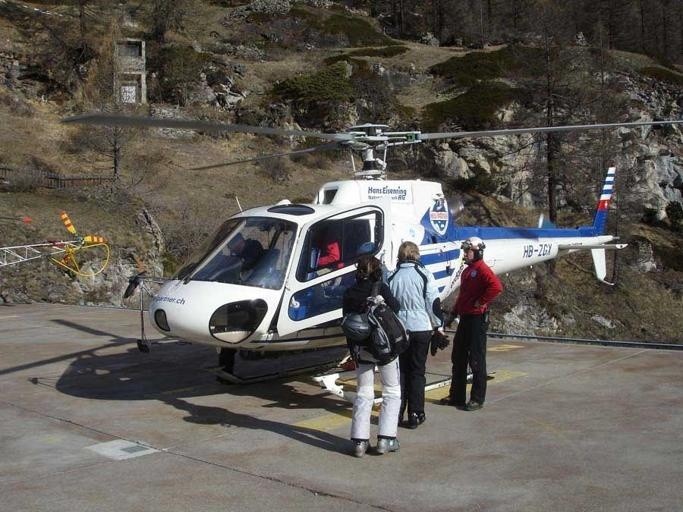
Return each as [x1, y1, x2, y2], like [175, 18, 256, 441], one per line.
[430, 330, 449, 356]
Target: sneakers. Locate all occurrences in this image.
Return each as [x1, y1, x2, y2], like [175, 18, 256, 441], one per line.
[350, 437, 370, 457]
[374, 436, 400, 454]
[464, 400, 482, 411]
[440, 394, 466, 406]
[398, 410, 426, 429]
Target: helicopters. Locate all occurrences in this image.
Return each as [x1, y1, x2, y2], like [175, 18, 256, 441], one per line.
[65, 115, 682, 386]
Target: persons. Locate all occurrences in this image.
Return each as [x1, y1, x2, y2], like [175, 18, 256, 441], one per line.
[341, 254, 400, 457]
[383, 240, 441, 425]
[308, 225, 345, 272]
[439, 236, 501, 411]
[224, 230, 268, 270]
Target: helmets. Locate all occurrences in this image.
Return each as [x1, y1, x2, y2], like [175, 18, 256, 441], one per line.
[460, 237, 486, 250]
[340, 312, 372, 342]
[398, 241, 420, 261]
[355, 241, 376, 256]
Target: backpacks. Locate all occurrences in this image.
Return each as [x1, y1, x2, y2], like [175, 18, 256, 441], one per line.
[367, 281, 410, 366]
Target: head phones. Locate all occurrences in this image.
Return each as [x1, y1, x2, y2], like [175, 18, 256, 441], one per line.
[476, 243, 485, 260]
